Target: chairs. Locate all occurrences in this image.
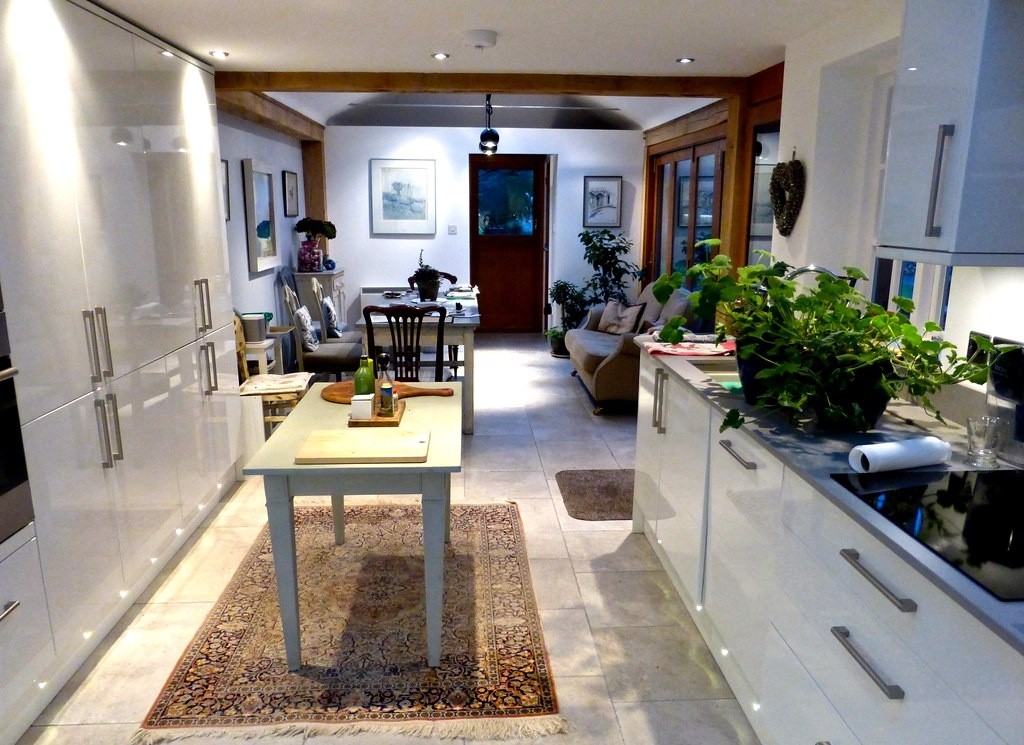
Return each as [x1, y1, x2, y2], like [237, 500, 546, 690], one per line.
[233, 278, 446, 440]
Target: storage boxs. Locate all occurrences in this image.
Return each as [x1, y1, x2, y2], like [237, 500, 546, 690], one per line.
[351, 393, 375, 420]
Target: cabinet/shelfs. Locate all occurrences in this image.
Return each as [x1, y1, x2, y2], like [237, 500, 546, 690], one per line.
[632, 350, 711, 632]
[701, 406, 784, 744]
[757, 464, 1023, 744]
[292, 267, 347, 328]
[0, 0, 245, 745]
[876, 0, 1023, 266]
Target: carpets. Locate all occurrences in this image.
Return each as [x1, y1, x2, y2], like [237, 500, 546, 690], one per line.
[129, 468, 632, 744]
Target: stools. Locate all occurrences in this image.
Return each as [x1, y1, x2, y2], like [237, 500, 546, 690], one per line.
[245, 339, 284, 376]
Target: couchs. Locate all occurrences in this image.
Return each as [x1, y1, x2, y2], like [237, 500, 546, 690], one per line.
[565, 282, 703, 416]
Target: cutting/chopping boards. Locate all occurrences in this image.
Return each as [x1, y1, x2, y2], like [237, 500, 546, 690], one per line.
[321, 379, 454, 403]
[295, 426, 431, 462]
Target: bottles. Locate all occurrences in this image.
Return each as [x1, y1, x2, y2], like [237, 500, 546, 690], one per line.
[354, 355, 374, 394]
[377, 366, 394, 417]
[392, 388, 399, 411]
[297, 241, 323, 273]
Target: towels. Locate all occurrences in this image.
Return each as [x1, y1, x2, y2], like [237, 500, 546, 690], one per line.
[652, 330, 736, 344]
[642, 340, 737, 356]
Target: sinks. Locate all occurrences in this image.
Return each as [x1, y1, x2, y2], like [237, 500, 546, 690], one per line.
[692, 363, 738, 374]
[707, 373, 740, 383]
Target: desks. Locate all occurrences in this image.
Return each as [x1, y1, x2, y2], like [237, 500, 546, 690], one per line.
[243, 382, 463, 672]
[355, 292, 480, 434]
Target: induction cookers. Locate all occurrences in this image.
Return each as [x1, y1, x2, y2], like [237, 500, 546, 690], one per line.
[829, 470, 1024, 602]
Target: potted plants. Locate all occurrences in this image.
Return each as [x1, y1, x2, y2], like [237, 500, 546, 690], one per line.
[294, 217, 337, 272]
[652, 238, 1021, 438]
[543, 228, 648, 355]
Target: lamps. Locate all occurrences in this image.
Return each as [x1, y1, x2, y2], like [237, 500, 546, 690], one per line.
[479, 94, 499, 156]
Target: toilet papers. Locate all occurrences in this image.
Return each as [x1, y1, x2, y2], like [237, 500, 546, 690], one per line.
[848, 436, 952, 474]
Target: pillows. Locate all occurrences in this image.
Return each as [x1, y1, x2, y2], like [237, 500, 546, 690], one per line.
[322, 296, 342, 338]
[636, 320, 660, 334]
[598, 297, 647, 335]
[294, 305, 320, 352]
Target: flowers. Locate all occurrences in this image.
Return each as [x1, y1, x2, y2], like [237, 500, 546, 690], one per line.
[412, 249, 444, 287]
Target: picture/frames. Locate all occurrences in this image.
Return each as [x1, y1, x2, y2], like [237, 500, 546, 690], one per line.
[221, 159, 299, 272]
[371, 158, 624, 234]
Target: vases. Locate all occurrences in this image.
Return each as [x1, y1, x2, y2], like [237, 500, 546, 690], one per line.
[418, 286, 439, 301]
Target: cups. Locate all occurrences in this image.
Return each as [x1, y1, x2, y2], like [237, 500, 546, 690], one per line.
[966, 416, 1008, 468]
